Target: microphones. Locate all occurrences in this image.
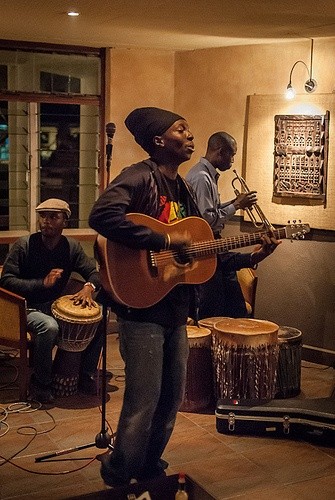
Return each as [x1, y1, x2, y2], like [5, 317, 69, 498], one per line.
[106, 123, 115, 158]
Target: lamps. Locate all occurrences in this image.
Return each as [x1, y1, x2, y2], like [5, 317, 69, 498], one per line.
[286, 61, 317, 99]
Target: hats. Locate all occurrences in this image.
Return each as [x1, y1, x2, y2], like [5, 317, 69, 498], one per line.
[35, 198, 71, 219]
[124, 107, 186, 153]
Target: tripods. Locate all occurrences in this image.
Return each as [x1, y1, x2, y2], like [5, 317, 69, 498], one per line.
[34, 303, 118, 462]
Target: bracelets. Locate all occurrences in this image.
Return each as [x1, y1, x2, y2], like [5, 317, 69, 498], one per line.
[165, 232, 172, 250]
[250, 251, 258, 270]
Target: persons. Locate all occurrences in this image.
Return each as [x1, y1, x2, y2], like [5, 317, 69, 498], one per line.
[0, 198, 109, 404]
[88, 107, 282, 489]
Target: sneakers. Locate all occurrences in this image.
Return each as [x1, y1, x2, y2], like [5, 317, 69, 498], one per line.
[29, 373, 57, 404]
[79, 373, 100, 395]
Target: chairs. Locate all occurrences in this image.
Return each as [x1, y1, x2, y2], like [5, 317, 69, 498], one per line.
[235, 267, 258, 318]
[0, 266, 32, 401]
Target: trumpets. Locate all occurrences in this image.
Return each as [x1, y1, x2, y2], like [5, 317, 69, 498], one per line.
[231, 169, 276, 232]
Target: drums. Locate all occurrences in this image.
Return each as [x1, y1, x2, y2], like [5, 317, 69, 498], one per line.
[198, 317, 230, 330]
[278, 326, 302, 398]
[50, 295, 103, 398]
[178, 325, 212, 412]
[212, 318, 279, 404]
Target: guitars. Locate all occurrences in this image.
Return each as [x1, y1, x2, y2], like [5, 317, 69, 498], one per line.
[95, 212, 311, 308]
